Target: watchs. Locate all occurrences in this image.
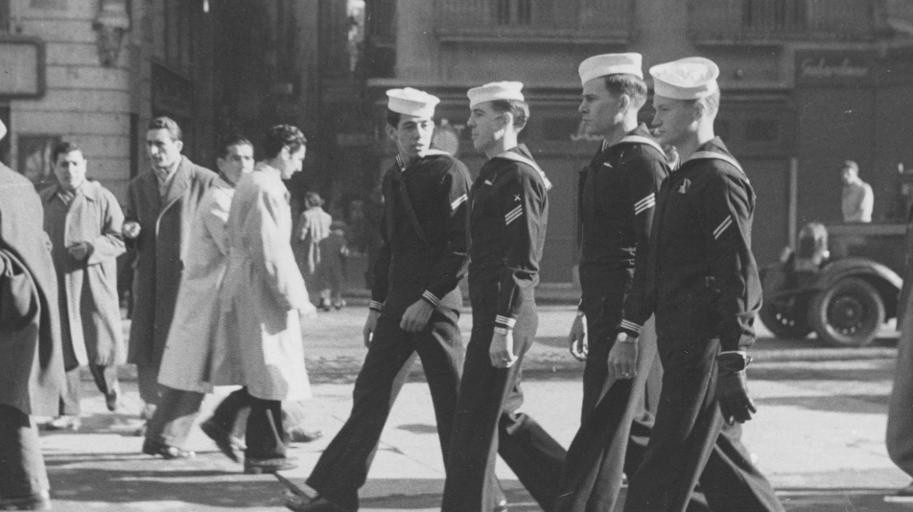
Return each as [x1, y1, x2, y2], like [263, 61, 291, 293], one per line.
[615, 331, 641, 346]
[716, 353, 752, 372]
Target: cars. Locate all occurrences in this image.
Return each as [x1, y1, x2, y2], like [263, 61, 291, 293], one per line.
[757, 166, 911, 350]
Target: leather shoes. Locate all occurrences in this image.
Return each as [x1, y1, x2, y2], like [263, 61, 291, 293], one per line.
[135, 414, 322, 473]
[324, 305, 331, 311]
[47, 416, 82, 431]
[284, 493, 350, 512]
[104, 379, 119, 411]
[334, 299, 346, 310]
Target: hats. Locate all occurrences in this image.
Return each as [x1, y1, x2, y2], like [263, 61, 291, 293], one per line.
[328, 220, 348, 232]
[578, 52, 645, 85]
[839, 160, 860, 172]
[467, 81, 526, 111]
[648, 55, 720, 100]
[386, 87, 441, 117]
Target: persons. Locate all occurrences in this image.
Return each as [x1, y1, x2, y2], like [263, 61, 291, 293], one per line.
[838, 160, 877, 223]
[1, 109, 366, 510]
[622, 54, 789, 512]
[436, 79, 571, 512]
[284, 85, 509, 512]
[543, 50, 707, 512]
